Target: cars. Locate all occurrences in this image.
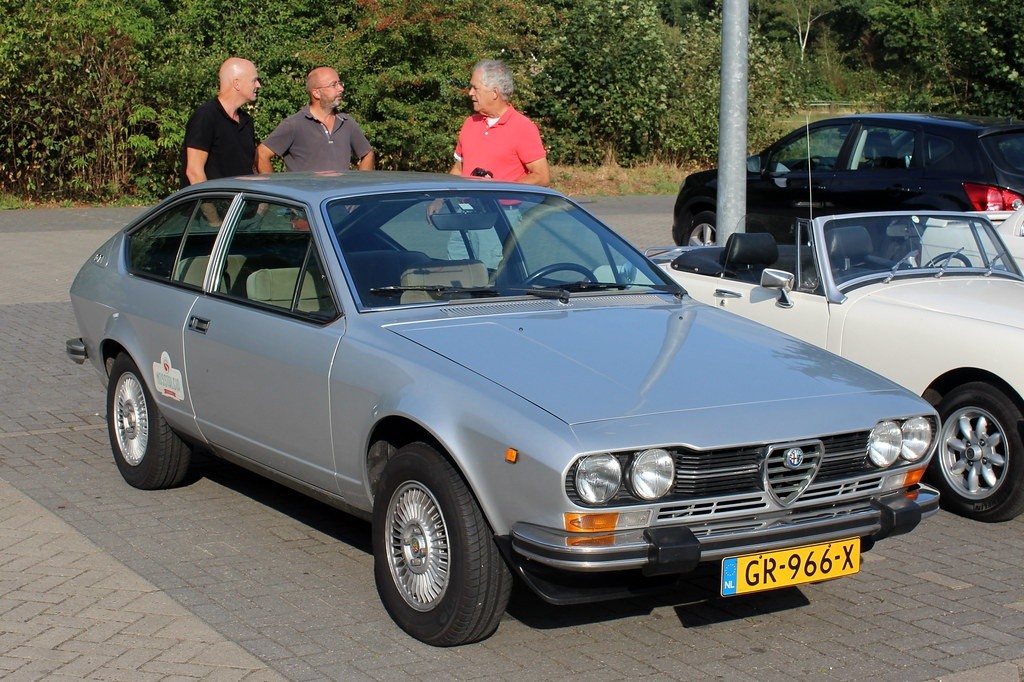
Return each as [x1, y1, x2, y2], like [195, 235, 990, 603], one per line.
[63, 163, 943, 649]
[917, 199, 1024, 285]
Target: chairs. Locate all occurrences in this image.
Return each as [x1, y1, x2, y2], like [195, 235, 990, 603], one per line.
[725, 233, 779, 284]
[864, 132, 897, 159]
[827, 225, 872, 270]
[176, 250, 490, 313]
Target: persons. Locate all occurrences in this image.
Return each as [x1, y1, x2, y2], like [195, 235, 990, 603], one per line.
[179, 56, 269, 230]
[254, 68, 374, 230]
[426, 59, 549, 270]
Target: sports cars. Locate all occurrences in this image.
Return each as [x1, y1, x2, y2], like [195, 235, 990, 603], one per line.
[585, 208, 1024, 523]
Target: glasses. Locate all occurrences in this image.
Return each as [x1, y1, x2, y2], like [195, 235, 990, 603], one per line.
[318, 81, 344, 89]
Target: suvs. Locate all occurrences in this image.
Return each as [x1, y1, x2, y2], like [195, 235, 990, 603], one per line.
[669, 113, 1024, 252]
